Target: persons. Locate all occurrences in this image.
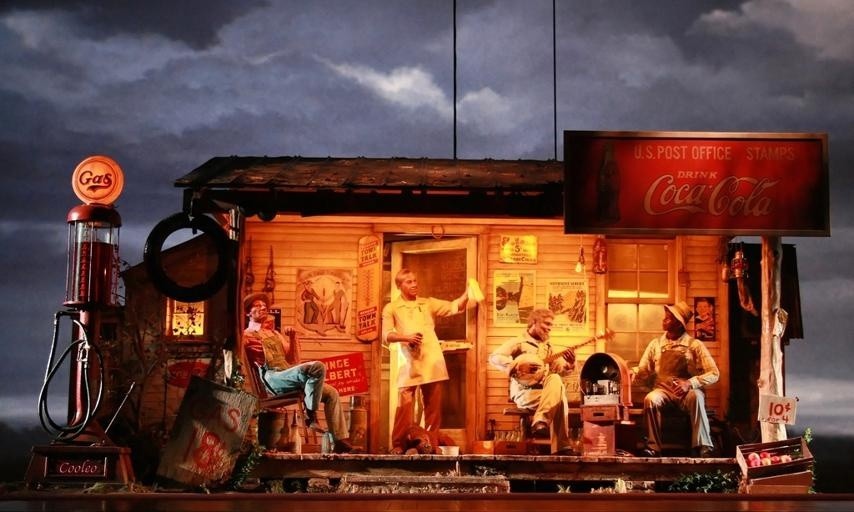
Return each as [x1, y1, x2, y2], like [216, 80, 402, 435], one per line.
[377, 267, 477, 457]
[239, 292, 366, 454]
[695, 297, 714, 337]
[630, 300, 720, 458]
[486, 307, 582, 457]
[324, 281, 349, 324]
[300, 279, 322, 325]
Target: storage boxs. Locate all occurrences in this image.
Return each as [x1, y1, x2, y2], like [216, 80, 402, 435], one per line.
[735, 435, 815, 491]
[577, 404, 630, 456]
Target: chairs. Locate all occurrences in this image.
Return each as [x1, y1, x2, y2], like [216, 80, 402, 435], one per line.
[241, 343, 319, 445]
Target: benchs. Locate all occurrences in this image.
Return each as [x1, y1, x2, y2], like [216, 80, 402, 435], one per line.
[502, 404, 712, 454]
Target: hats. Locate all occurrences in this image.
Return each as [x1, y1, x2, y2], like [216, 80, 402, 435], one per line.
[663, 300, 694, 333]
[244, 292, 271, 310]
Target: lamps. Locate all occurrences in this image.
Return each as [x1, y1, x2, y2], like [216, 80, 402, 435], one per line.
[49, 203, 120, 445]
[575, 246, 583, 272]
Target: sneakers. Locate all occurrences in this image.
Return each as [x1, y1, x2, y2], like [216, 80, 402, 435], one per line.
[642, 449, 660, 458]
[531, 422, 550, 434]
[700, 445, 715, 458]
[331, 441, 365, 454]
[303, 407, 324, 433]
[551, 448, 583, 456]
